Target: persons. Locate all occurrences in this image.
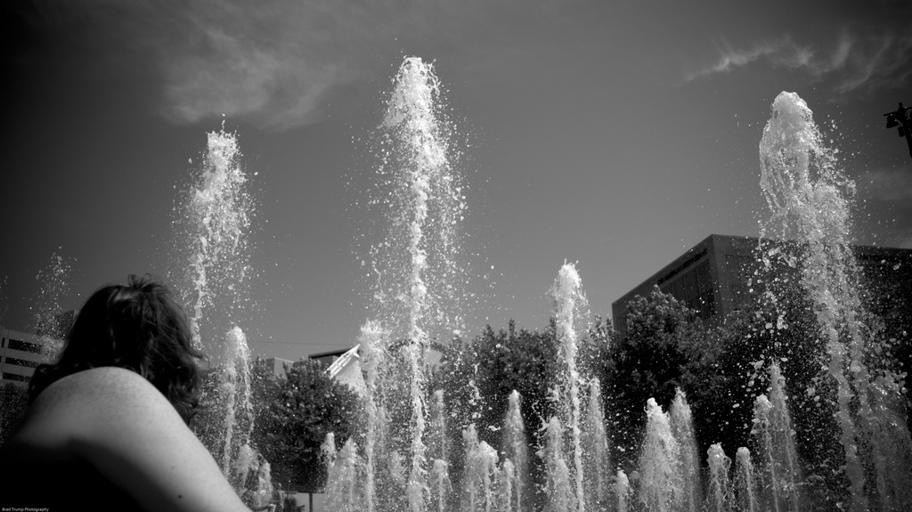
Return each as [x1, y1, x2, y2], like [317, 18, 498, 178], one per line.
[0, 273, 254, 512]
